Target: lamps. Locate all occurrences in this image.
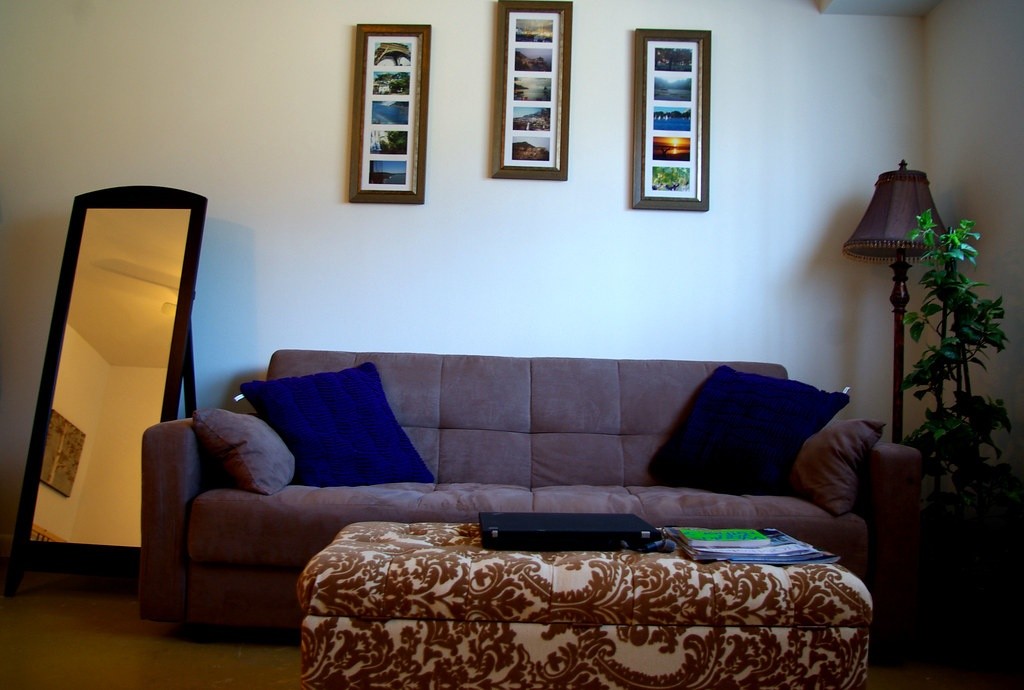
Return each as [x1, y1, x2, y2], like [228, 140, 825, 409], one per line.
[841, 157, 947, 445]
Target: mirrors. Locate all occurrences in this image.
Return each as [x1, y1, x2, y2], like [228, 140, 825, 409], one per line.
[0, 185, 208, 600]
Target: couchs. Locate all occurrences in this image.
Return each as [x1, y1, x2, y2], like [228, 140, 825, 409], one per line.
[140, 349, 924, 667]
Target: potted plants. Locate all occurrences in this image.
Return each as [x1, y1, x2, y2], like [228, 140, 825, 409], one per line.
[899, 207, 1024, 673]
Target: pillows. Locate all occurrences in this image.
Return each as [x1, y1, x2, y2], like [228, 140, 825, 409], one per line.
[648, 365, 850, 494]
[240, 363, 435, 487]
[790, 419, 887, 517]
[192, 407, 294, 496]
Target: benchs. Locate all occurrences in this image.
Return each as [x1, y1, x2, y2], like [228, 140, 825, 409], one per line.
[297, 519, 873, 690]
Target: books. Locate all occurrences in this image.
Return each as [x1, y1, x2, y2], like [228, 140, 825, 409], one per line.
[655, 527, 841, 566]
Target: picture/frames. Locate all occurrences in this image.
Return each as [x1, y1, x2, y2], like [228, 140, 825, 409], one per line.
[349, 23, 432, 205]
[491, 0, 573, 182]
[632, 28, 713, 212]
